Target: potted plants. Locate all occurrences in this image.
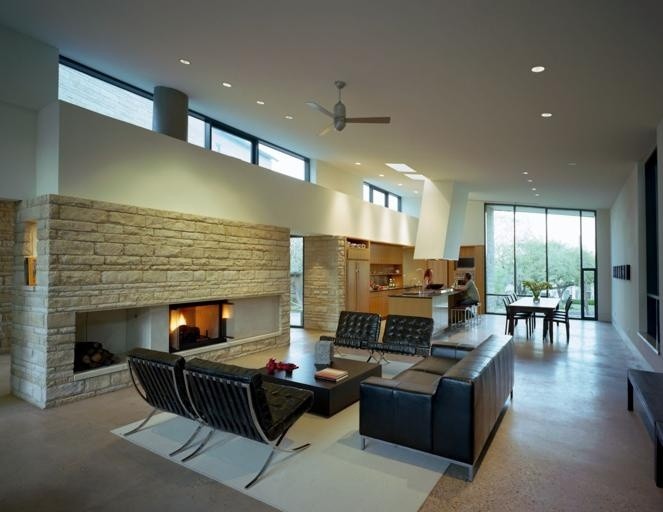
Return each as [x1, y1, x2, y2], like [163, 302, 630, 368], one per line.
[521, 278, 553, 303]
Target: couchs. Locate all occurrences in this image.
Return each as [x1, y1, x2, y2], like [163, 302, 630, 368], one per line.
[357, 334, 514, 482]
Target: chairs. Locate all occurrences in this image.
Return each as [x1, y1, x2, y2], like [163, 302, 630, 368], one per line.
[123, 344, 202, 457]
[502, 292, 572, 345]
[367, 314, 434, 371]
[183, 351, 311, 487]
[318, 311, 381, 367]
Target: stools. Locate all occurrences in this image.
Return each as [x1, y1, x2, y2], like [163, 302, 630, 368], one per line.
[450, 300, 482, 331]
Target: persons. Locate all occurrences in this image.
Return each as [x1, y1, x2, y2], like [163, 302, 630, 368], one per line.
[423, 268, 433, 286]
[453, 273, 480, 319]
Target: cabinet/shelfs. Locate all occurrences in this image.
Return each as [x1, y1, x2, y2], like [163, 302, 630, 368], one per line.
[343, 237, 370, 312]
[303, 80, 391, 139]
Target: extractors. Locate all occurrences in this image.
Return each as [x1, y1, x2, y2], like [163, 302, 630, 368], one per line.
[413, 180, 470, 261]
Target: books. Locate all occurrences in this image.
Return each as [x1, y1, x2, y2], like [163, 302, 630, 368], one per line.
[315, 368, 349, 382]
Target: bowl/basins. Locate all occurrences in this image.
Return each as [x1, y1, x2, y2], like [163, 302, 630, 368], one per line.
[429, 284, 444, 289]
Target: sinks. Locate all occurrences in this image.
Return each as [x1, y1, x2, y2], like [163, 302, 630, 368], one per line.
[401, 292, 432, 294]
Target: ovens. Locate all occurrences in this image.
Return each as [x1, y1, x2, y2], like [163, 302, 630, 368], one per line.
[457, 257, 475, 271]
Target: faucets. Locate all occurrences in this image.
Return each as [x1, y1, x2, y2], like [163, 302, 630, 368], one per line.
[416, 268, 425, 291]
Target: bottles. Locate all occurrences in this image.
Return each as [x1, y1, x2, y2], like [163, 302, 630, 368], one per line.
[389, 278, 396, 287]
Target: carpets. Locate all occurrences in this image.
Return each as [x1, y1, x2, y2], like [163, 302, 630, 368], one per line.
[108, 351, 450, 512]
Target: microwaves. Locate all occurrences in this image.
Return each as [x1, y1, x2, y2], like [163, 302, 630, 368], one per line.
[455, 277, 468, 286]
